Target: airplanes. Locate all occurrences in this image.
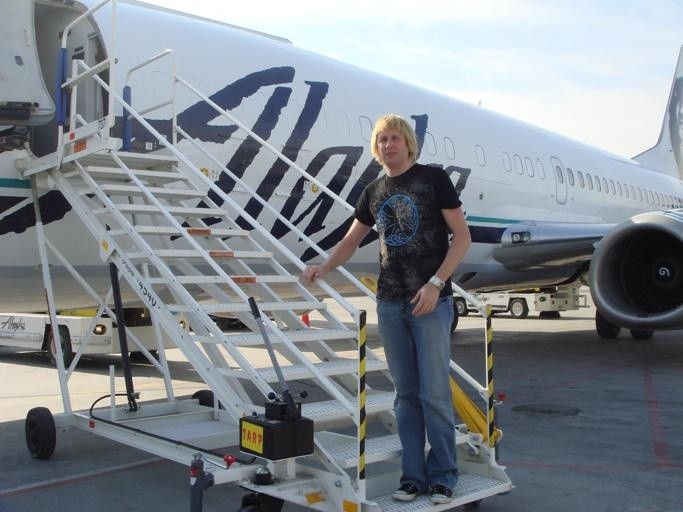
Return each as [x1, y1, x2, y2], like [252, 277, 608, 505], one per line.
[0, 0, 683, 342]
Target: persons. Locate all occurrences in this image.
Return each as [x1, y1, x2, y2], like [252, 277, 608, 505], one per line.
[302, 114, 472, 504]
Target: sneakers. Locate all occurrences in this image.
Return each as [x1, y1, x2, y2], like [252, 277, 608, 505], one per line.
[431, 486, 452, 503]
[392, 483, 420, 500]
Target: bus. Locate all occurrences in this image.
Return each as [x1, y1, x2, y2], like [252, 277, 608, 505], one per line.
[452, 276, 590, 318]
[0, 308, 190, 367]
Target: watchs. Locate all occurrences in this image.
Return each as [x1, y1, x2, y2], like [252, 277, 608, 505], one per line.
[429, 275, 445, 290]
